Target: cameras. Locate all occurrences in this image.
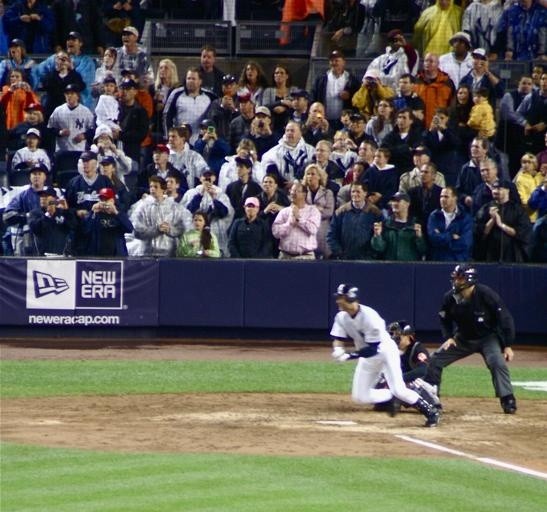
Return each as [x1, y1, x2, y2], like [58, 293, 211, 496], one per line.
[433, 117, 439, 124]
[49, 200, 58, 212]
[316, 113, 322, 122]
[15, 81, 25, 88]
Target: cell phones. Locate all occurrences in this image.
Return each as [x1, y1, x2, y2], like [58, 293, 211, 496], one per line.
[481, 57, 486, 65]
[208, 126, 215, 135]
[224, 88, 232, 96]
[257, 119, 265, 134]
[206, 174, 211, 183]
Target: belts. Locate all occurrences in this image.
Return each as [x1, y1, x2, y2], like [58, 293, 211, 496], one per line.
[280, 250, 310, 256]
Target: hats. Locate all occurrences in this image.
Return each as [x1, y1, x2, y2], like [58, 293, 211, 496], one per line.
[151, 144, 169, 153]
[449, 32, 472, 47]
[290, 90, 309, 98]
[200, 75, 271, 127]
[350, 113, 365, 122]
[411, 145, 429, 154]
[64, 25, 139, 92]
[472, 48, 487, 59]
[328, 49, 342, 59]
[390, 191, 409, 201]
[200, 156, 260, 207]
[26, 124, 115, 200]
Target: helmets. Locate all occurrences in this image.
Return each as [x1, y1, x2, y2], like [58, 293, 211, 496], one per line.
[332, 284, 359, 301]
[387, 320, 416, 341]
[450, 264, 479, 294]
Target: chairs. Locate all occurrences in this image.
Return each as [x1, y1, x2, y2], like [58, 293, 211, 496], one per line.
[0, 125, 166, 204]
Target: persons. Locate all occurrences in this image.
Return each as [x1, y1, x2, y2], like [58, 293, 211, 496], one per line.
[429, 262, 517, 413]
[375, 320, 430, 410]
[1, 1, 545, 259]
[330, 284, 440, 428]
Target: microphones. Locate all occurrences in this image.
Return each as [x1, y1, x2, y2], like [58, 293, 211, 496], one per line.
[103, 203, 110, 209]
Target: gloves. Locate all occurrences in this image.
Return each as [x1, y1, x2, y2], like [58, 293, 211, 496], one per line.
[332, 347, 350, 361]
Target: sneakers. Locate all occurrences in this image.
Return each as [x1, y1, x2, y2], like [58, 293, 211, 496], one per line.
[501, 394, 516, 414]
[371, 384, 443, 427]
